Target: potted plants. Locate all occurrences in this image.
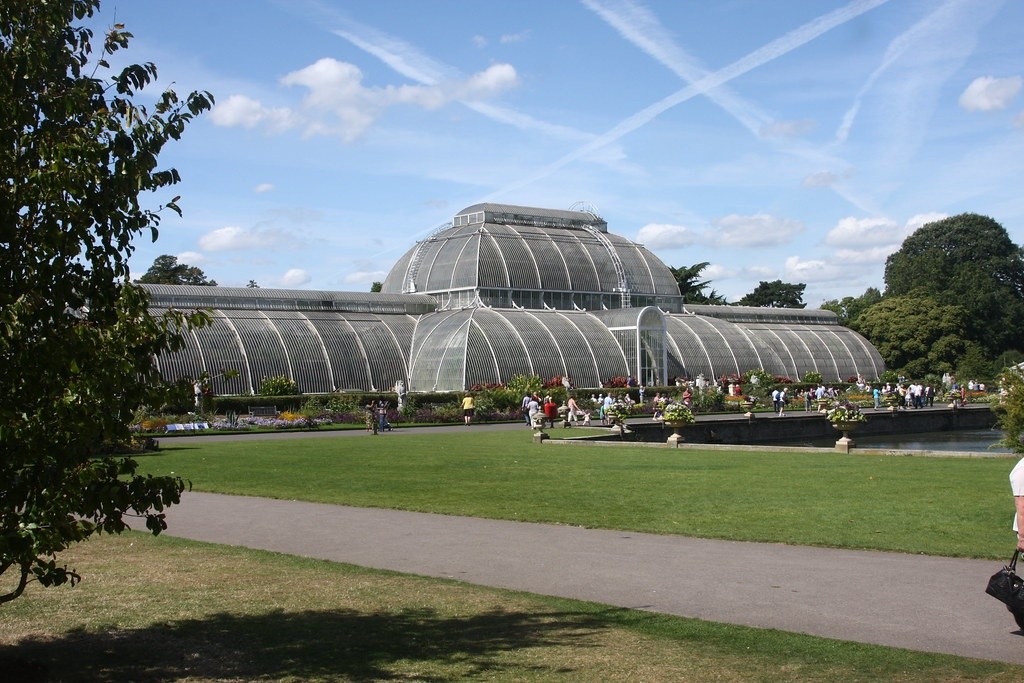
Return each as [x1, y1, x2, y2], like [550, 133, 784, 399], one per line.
[817, 397, 830, 413]
[885, 391, 902, 411]
[606, 403, 629, 431]
[742, 396, 760, 416]
[662, 403, 695, 441]
[944, 389, 962, 407]
[826, 400, 869, 445]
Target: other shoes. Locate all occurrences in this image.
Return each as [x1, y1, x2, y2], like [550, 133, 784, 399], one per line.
[1007, 604, 1024, 629]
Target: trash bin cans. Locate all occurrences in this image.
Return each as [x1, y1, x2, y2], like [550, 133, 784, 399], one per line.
[544, 403, 558, 428]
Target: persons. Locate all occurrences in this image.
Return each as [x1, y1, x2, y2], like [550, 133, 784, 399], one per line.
[846, 372, 985, 411]
[985, 456, 1024, 630]
[560, 376, 647, 424]
[651, 373, 842, 422]
[521, 392, 552, 427]
[194, 379, 214, 411]
[460, 393, 475, 425]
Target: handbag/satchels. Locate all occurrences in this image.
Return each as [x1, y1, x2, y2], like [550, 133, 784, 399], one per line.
[985, 550, 1024, 608]
[522, 407, 529, 414]
